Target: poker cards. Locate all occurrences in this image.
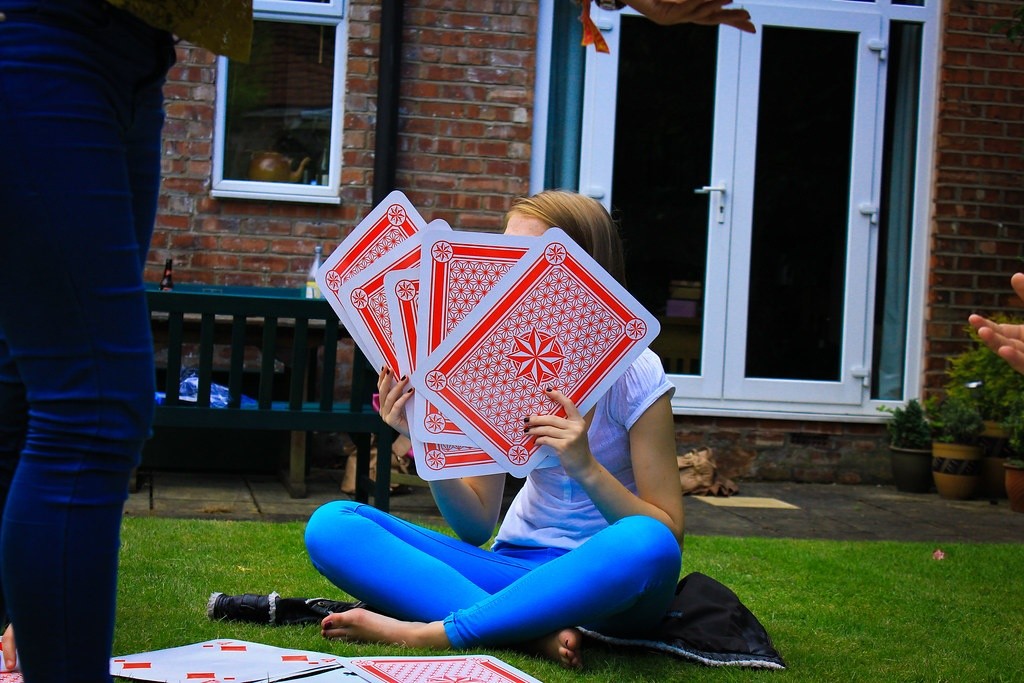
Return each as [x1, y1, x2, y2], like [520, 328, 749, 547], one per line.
[1, 636, 547, 683]
[311, 189, 663, 483]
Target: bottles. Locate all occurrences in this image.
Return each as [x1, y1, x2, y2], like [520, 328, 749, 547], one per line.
[210, 267, 226, 284]
[320, 147, 328, 185]
[259, 266, 273, 286]
[159, 259, 174, 291]
[306, 246, 324, 299]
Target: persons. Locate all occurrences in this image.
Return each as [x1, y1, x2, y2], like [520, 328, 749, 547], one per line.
[300, 188, 686, 668]
[965, 272, 1024, 376]
[2, 623, 20, 670]
[0, 0, 759, 682]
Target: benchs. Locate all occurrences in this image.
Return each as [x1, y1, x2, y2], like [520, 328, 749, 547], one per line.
[143, 283, 396, 516]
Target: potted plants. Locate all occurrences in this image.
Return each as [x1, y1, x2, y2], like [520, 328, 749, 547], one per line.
[877, 309, 1024, 512]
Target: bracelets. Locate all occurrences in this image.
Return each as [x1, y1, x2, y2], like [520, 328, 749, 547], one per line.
[592, 0, 625, 12]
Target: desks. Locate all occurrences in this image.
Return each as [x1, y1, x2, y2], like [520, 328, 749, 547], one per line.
[149, 311, 347, 497]
[648, 317, 701, 374]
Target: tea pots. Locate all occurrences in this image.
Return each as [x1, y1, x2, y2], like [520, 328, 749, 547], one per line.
[249, 150, 309, 182]
